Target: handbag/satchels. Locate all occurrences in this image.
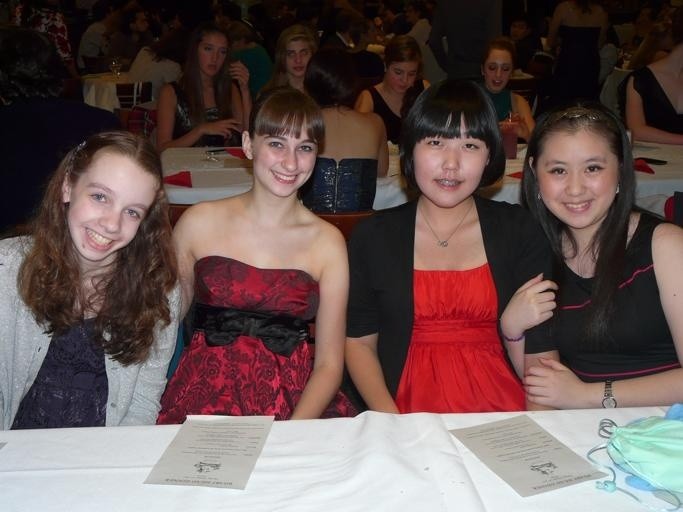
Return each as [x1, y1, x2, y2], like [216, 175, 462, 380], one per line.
[587, 402, 683, 511]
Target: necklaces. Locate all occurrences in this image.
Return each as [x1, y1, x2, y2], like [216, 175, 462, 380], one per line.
[417, 206, 478, 247]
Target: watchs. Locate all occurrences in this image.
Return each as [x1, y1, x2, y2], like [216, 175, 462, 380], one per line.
[604, 378, 616, 411]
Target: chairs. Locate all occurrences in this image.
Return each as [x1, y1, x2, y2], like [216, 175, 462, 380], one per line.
[511, 78, 540, 110]
[115, 82, 152, 108]
[314, 210, 374, 241]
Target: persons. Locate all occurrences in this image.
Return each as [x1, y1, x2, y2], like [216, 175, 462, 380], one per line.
[355, 36, 434, 145]
[155, 86, 356, 425]
[106, 6, 153, 72]
[506, 19, 543, 73]
[76, 3, 131, 76]
[0, 132, 183, 429]
[472, 35, 536, 142]
[154, 28, 252, 153]
[548, 0, 615, 107]
[125, 30, 189, 101]
[0, 27, 118, 225]
[496, 102, 683, 409]
[342, 79, 559, 414]
[160, 1, 500, 34]
[256, 27, 317, 102]
[625, 13, 683, 145]
[0, 0, 84, 106]
[302, 48, 391, 217]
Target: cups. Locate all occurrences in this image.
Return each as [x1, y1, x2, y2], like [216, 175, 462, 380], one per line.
[111, 63, 121, 78]
